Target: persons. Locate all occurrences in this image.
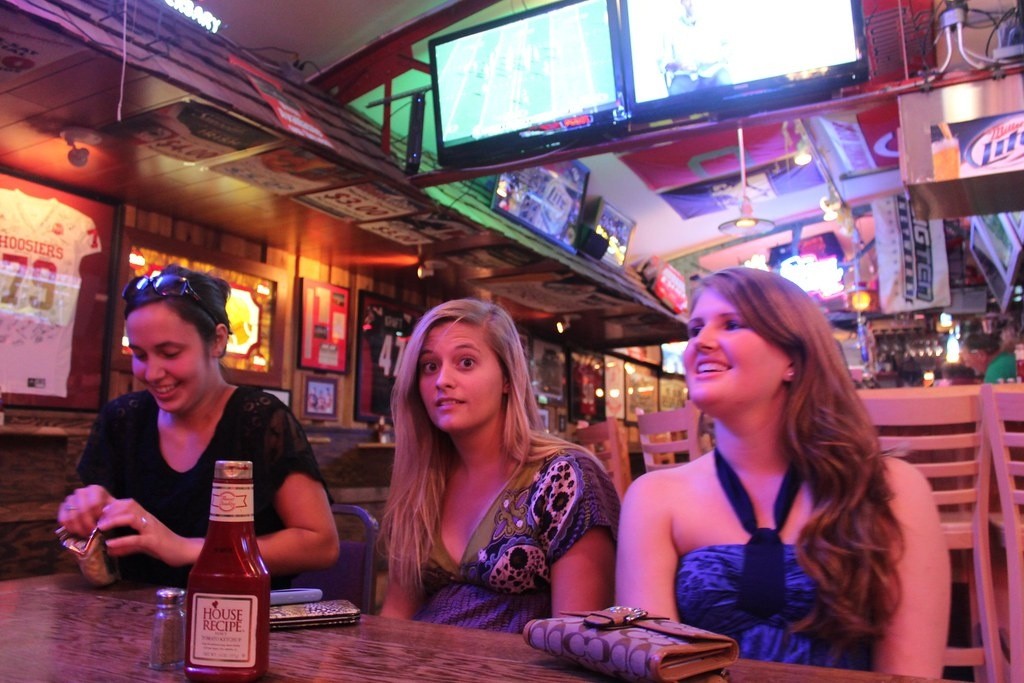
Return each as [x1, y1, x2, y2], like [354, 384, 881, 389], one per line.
[373, 297, 621, 639]
[54, 266, 339, 597]
[958, 328, 1020, 385]
[613, 265, 951, 681]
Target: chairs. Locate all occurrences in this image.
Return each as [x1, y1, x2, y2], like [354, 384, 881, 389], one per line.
[855, 391, 1009, 683]
[316, 443, 398, 505]
[576, 420, 632, 504]
[980, 383, 1024, 683]
[637, 398, 703, 472]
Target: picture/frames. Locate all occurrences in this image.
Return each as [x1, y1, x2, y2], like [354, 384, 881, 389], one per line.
[604, 352, 625, 421]
[353, 289, 427, 426]
[296, 277, 353, 374]
[111, 225, 288, 388]
[624, 361, 659, 427]
[514, 327, 530, 361]
[299, 372, 341, 421]
[567, 345, 606, 424]
[976, 213, 1022, 286]
[262, 387, 292, 409]
[967, 216, 1013, 313]
[530, 338, 568, 408]
[658, 371, 689, 410]
[490, 158, 591, 257]
[1007, 210, 1024, 246]
[0, 166, 124, 411]
[533, 404, 555, 433]
[559, 416, 566, 432]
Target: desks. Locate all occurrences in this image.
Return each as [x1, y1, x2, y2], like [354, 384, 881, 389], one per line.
[0, 569, 956, 683]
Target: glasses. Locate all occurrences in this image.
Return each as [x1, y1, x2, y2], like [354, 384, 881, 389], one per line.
[123, 276, 220, 327]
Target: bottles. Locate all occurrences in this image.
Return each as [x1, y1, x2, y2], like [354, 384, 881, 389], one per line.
[148, 587, 185, 670]
[184, 461, 271, 683]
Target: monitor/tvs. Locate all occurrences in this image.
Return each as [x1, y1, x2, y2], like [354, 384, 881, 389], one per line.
[428, 0, 628, 166]
[619, 0, 868, 124]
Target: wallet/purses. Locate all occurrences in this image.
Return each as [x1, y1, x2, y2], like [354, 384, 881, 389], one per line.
[523, 607, 739, 682]
[268, 598, 362, 629]
[64, 527, 120, 587]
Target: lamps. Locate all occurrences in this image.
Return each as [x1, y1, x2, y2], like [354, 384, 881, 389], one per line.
[416, 261, 446, 278]
[556, 315, 581, 333]
[718, 129, 775, 235]
[819, 193, 856, 237]
[790, 135, 815, 166]
[58, 126, 98, 169]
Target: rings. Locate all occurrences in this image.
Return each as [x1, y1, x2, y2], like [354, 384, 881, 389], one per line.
[140, 517, 148, 530]
[65, 503, 76, 512]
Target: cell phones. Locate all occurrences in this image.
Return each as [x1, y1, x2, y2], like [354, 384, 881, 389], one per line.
[269, 588, 323, 606]
